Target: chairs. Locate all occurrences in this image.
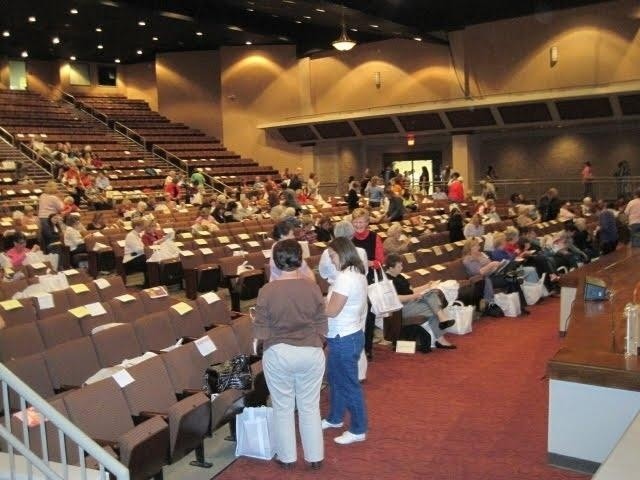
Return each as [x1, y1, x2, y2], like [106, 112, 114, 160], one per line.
[168, 300, 206, 344]
[0, 398, 119, 470]
[63, 376, 169, 480]
[180, 248, 272, 300]
[159, 341, 244, 441]
[0, 87, 282, 248]
[297, 193, 601, 341]
[139, 285, 172, 315]
[58, 267, 88, 286]
[0, 279, 29, 302]
[91, 322, 142, 368]
[0, 297, 37, 331]
[42, 335, 102, 394]
[86, 248, 180, 287]
[33, 290, 72, 320]
[96, 275, 128, 302]
[79, 301, 117, 336]
[66, 282, 101, 308]
[228, 314, 262, 356]
[195, 290, 241, 327]
[27, 273, 65, 293]
[0, 321, 47, 363]
[0, 353, 55, 416]
[36, 311, 84, 349]
[133, 310, 177, 355]
[205, 326, 263, 391]
[121, 356, 213, 468]
[109, 292, 146, 323]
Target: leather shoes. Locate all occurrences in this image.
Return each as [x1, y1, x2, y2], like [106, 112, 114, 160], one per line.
[434, 340, 456, 349]
[438, 319, 456, 330]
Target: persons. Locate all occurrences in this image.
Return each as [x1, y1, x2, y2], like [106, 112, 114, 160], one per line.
[447, 203, 465, 243]
[384, 188, 404, 222]
[543, 234, 582, 268]
[84, 177, 107, 211]
[186, 180, 199, 203]
[351, 208, 384, 360]
[63, 165, 84, 207]
[464, 193, 497, 238]
[122, 218, 145, 274]
[31, 134, 49, 163]
[95, 172, 110, 191]
[383, 224, 410, 256]
[308, 175, 321, 197]
[39, 181, 65, 254]
[65, 216, 91, 254]
[6, 232, 40, 267]
[507, 228, 561, 295]
[487, 164, 498, 190]
[141, 219, 166, 246]
[479, 179, 496, 199]
[430, 187, 447, 199]
[538, 187, 561, 223]
[191, 169, 205, 194]
[195, 206, 217, 227]
[212, 165, 290, 226]
[319, 220, 368, 285]
[51, 142, 101, 172]
[447, 172, 464, 202]
[488, 233, 514, 261]
[385, 165, 410, 187]
[462, 237, 532, 316]
[164, 171, 178, 185]
[385, 255, 456, 349]
[402, 188, 418, 211]
[582, 160, 594, 199]
[347, 176, 360, 214]
[516, 205, 540, 230]
[547, 223, 577, 251]
[167, 180, 181, 205]
[21, 205, 39, 227]
[321, 237, 368, 444]
[130, 201, 148, 224]
[269, 222, 315, 281]
[254, 239, 328, 470]
[62, 196, 82, 215]
[419, 167, 430, 195]
[365, 176, 383, 208]
[290, 167, 302, 189]
[43, 214, 72, 269]
[589, 204, 620, 255]
[624, 190, 640, 247]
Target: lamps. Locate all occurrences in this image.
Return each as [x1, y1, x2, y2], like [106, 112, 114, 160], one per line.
[330, 5, 358, 53]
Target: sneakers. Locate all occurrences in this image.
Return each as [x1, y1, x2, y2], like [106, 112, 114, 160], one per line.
[321, 419, 345, 430]
[332, 430, 367, 446]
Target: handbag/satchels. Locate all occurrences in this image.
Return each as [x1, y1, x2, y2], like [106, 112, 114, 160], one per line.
[367, 260, 404, 316]
[446, 300, 475, 335]
[493, 291, 522, 318]
[203, 354, 253, 398]
[232, 405, 276, 461]
[520, 271, 547, 306]
